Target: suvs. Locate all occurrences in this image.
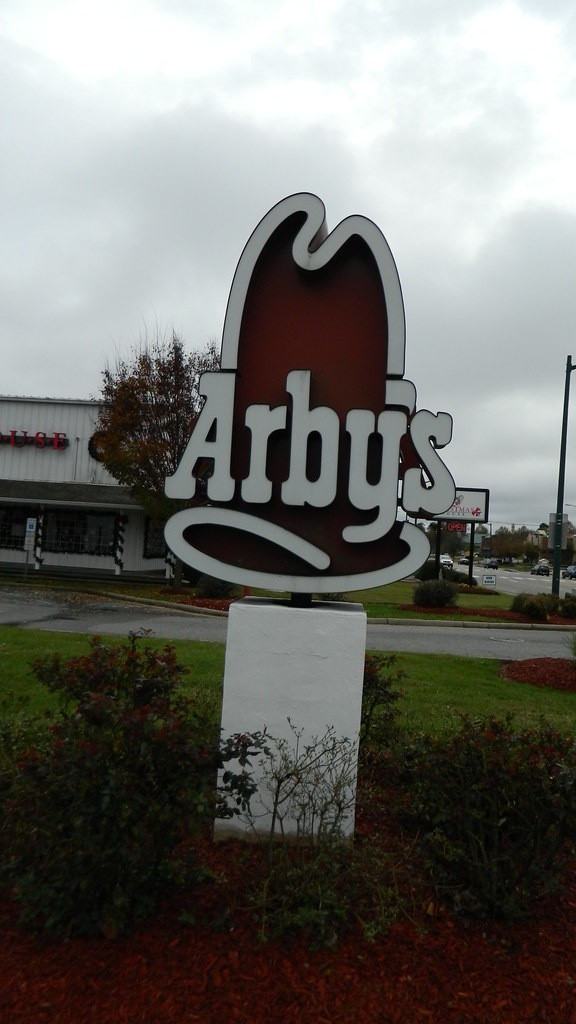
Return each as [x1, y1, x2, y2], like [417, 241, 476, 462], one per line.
[438, 555, 454, 568]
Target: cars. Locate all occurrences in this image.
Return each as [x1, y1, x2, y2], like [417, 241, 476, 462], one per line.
[428, 553, 437, 563]
[531, 564, 550, 577]
[458, 559, 469, 566]
[484, 560, 498, 571]
[562, 565, 576, 581]
